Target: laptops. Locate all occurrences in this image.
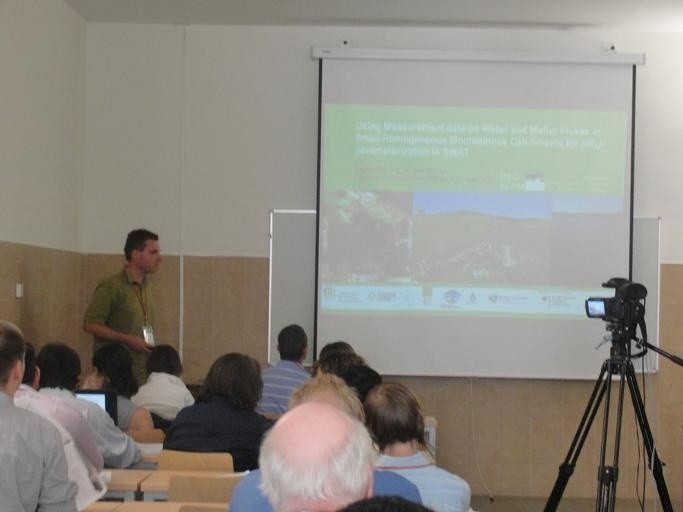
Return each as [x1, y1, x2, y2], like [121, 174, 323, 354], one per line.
[74, 389, 118, 429]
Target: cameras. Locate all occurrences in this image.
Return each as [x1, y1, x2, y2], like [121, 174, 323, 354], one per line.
[583, 277, 648, 323]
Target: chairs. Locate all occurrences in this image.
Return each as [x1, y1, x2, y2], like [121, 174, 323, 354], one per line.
[158, 450, 234, 472]
[129, 428, 165, 442]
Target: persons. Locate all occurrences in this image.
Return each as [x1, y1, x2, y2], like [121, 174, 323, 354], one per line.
[83, 229, 163, 386]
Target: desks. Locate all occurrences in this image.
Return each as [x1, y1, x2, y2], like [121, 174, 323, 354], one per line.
[138, 443, 164, 464]
[86, 468, 233, 510]
[424, 415, 437, 449]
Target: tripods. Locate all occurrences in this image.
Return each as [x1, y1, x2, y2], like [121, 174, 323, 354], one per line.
[541, 324, 683, 512]
